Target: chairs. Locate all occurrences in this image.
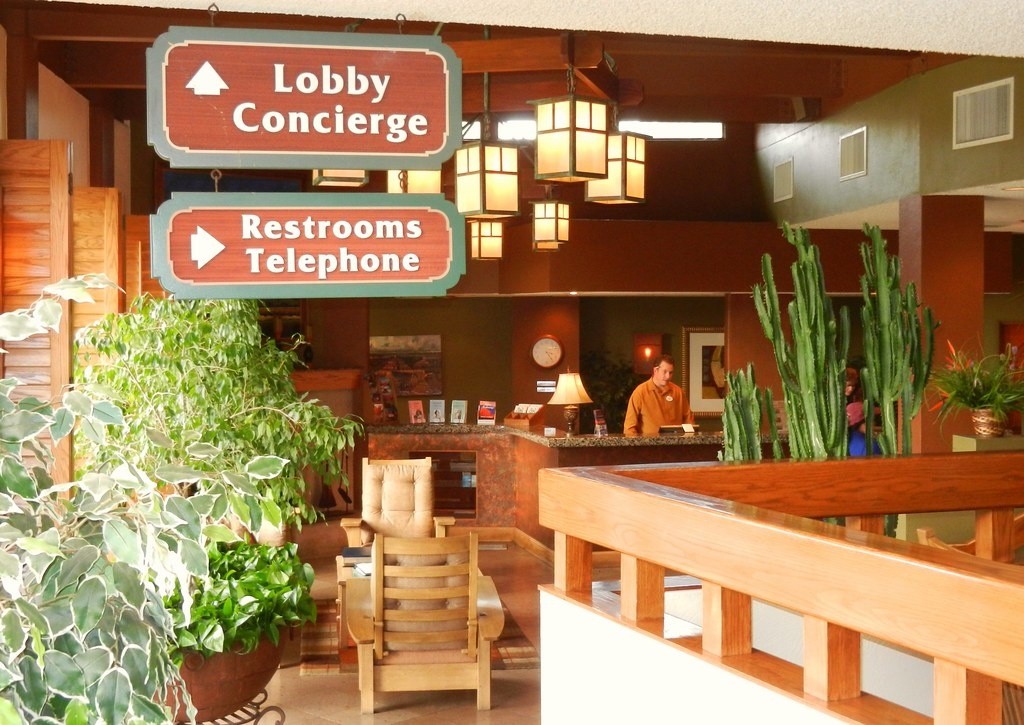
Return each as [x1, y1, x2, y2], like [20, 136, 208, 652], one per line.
[346, 530, 506, 714]
[341, 456, 455, 547]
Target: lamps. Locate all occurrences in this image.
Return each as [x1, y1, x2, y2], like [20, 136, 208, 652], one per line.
[454, 25, 529, 220]
[313, 169, 369, 188]
[528, 184, 575, 243]
[467, 218, 509, 260]
[583, 104, 652, 205]
[532, 242, 560, 253]
[524, 66, 613, 183]
[546, 365, 594, 436]
[634, 333, 662, 375]
[387, 169, 441, 193]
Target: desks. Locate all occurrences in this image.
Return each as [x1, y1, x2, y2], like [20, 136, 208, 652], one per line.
[336, 555, 483, 672]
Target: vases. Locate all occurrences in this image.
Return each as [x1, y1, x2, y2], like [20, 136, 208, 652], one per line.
[970, 408, 1007, 437]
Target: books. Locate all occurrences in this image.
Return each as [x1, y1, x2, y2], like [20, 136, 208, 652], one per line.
[477, 401, 497, 425]
[450, 400, 468, 424]
[429, 400, 445, 422]
[408, 400, 425, 423]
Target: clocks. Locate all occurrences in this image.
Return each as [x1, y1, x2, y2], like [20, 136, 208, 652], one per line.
[529, 333, 565, 370]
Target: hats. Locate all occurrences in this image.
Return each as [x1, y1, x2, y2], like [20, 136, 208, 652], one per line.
[847, 402, 881, 426]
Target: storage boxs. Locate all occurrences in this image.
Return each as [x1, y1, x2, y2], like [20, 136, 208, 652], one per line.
[477, 401, 496, 425]
[504, 403, 546, 426]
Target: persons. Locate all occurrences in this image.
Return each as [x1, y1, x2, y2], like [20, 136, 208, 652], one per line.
[623, 354, 694, 437]
[844, 367, 884, 457]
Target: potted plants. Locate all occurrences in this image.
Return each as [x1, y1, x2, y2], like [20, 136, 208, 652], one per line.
[0, 294, 366, 725]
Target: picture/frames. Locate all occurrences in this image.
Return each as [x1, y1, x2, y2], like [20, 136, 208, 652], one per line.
[681, 326, 727, 418]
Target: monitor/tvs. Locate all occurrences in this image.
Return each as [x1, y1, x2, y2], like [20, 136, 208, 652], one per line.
[658, 425, 699, 433]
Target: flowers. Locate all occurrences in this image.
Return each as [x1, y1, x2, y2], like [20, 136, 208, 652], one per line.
[919, 337, 1024, 448]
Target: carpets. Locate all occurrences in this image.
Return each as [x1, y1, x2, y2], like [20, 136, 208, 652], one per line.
[299, 597, 542, 678]
